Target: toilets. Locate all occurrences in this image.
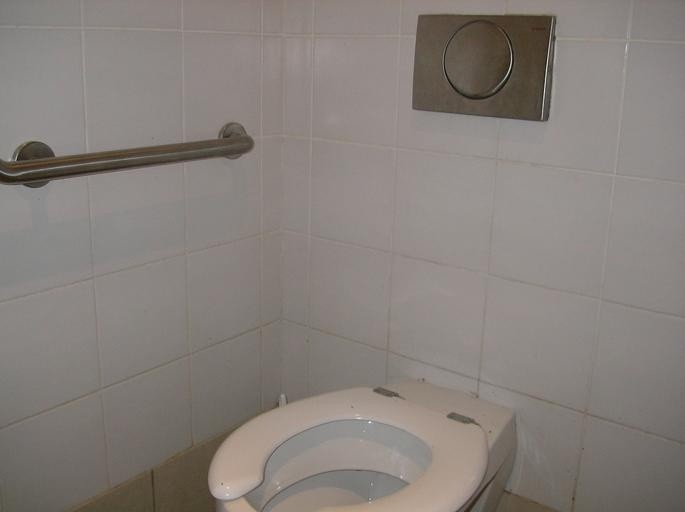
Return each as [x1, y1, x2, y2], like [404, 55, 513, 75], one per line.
[206, 379, 518, 512]
